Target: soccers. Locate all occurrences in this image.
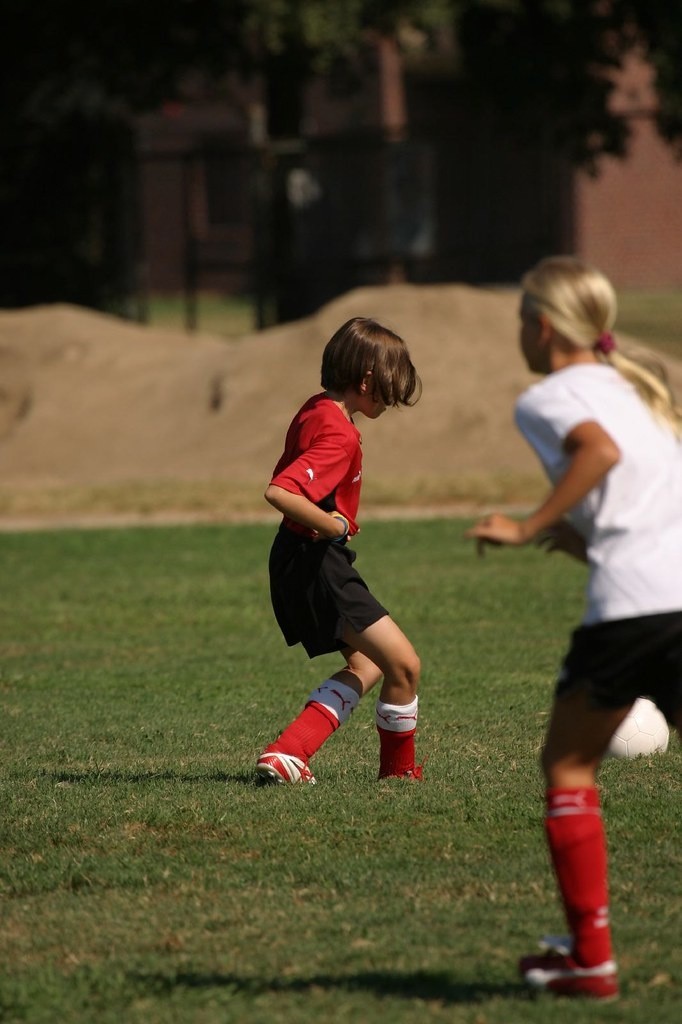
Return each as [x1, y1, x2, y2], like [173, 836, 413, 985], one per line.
[605, 692, 673, 767]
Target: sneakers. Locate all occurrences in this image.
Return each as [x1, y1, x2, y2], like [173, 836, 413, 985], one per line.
[379, 764, 430, 782]
[258, 743, 318, 785]
[515, 947, 622, 1002]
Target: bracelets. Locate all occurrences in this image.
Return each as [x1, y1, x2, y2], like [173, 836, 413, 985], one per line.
[332, 515, 350, 541]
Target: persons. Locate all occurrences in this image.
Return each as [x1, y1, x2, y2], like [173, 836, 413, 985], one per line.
[255, 318, 422, 784]
[461, 256, 682, 1003]
